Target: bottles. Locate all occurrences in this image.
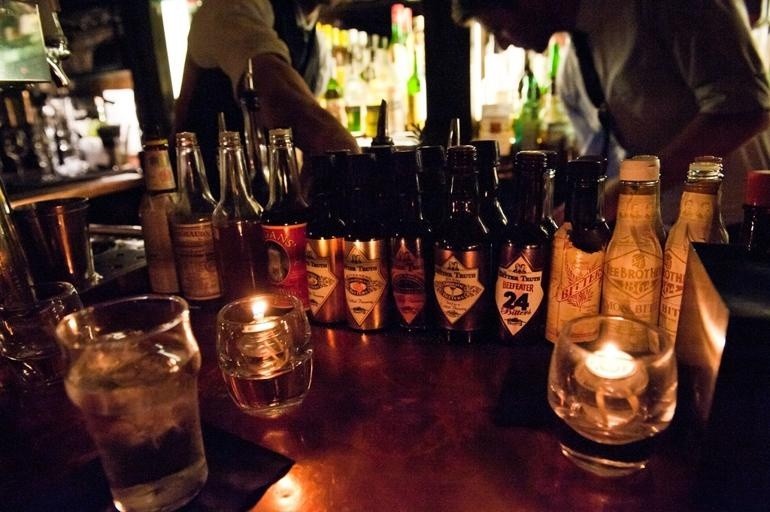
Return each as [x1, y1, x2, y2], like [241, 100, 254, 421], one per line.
[0, 76, 137, 189]
[141, 128, 770, 340]
[241, 90, 271, 208]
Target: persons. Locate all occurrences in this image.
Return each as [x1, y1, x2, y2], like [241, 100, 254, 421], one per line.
[168, 0, 362, 206]
[449, 0, 770, 244]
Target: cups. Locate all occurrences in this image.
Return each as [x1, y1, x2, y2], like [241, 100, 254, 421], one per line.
[0, 278, 111, 470]
[0, 188, 42, 290]
[9, 194, 96, 293]
[213, 296, 314, 417]
[545, 313, 679, 479]
[56, 295, 212, 511]
